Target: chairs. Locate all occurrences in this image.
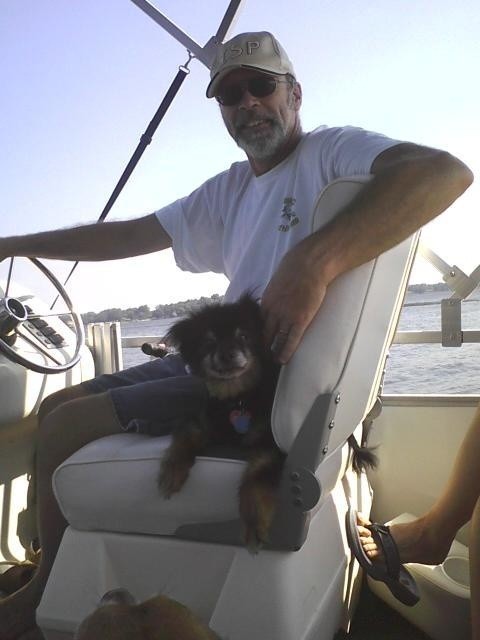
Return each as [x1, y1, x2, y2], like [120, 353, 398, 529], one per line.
[50, 174, 424, 555]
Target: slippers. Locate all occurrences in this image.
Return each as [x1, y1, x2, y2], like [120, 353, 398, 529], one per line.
[346, 509, 420, 607]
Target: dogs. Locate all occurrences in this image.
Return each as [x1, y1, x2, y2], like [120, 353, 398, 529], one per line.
[154, 286, 287, 557]
[73, 587, 224, 640]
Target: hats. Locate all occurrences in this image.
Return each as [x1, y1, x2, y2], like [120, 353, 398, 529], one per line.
[206, 31, 296, 98]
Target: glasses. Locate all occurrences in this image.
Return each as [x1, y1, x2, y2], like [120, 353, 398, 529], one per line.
[214, 73, 296, 106]
